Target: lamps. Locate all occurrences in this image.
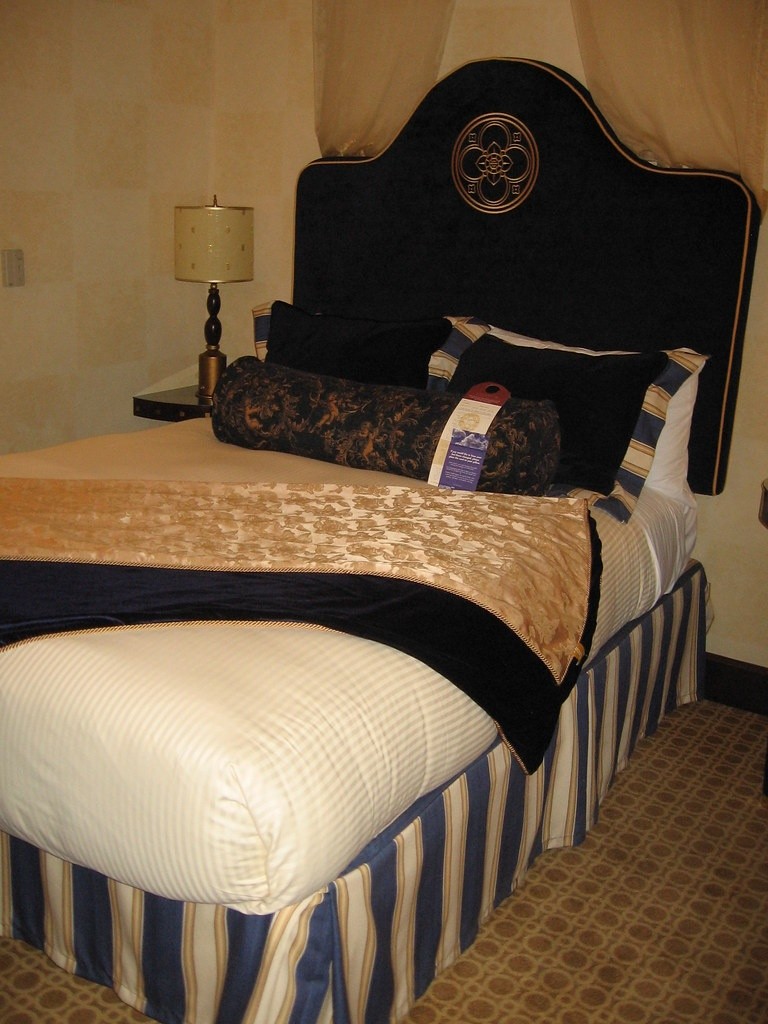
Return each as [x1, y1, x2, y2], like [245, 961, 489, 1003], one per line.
[174, 194, 255, 398]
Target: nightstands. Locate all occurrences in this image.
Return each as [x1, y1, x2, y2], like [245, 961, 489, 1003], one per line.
[133, 384, 215, 423]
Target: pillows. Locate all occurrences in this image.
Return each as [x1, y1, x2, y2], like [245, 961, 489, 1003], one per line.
[210, 355, 562, 496]
[251, 300, 492, 393]
[488, 326, 698, 507]
[450, 322, 711, 526]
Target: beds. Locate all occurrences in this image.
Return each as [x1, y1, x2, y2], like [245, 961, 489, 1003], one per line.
[0, 56, 759, 1024]
